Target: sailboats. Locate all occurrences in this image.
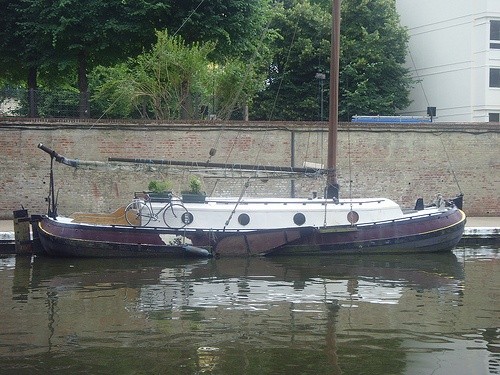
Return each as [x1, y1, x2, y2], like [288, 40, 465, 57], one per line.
[15, 0, 466, 257]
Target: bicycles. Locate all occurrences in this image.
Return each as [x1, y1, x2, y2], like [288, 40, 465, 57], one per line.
[125, 191, 190, 229]
